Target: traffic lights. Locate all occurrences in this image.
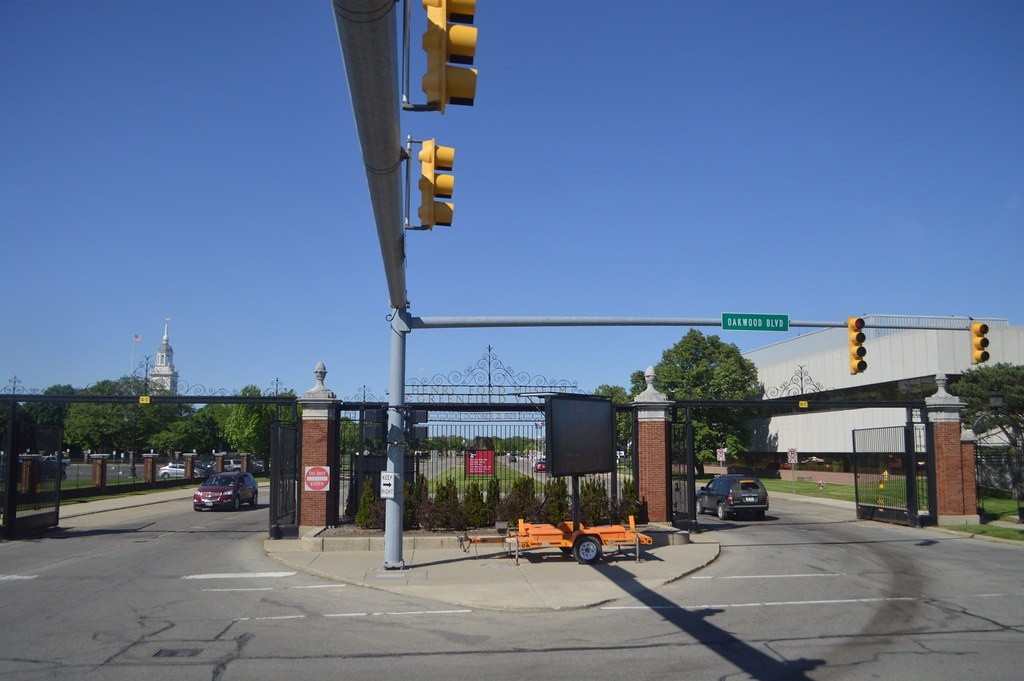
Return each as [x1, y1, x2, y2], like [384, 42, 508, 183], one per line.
[848, 317, 868, 375]
[400, 0, 478, 113]
[404, 135, 455, 232]
[971, 322, 991, 364]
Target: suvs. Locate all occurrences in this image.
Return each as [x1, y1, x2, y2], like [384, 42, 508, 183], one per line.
[696, 475, 768, 520]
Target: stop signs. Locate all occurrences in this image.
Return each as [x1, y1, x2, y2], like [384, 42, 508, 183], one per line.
[305, 466, 330, 492]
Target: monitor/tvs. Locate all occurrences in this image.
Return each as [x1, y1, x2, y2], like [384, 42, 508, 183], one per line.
[544, 394, 616, 478]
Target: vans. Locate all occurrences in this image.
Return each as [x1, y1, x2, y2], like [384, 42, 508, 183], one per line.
[213, 459, 242, 472]
[257, 461, 271, 468]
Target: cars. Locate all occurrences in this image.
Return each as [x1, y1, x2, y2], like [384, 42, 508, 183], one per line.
[159, 464, 209, 479]
[509, 456, 516, 462]
[534, 461, 547, 473]
[194, 472, 258, 510]
